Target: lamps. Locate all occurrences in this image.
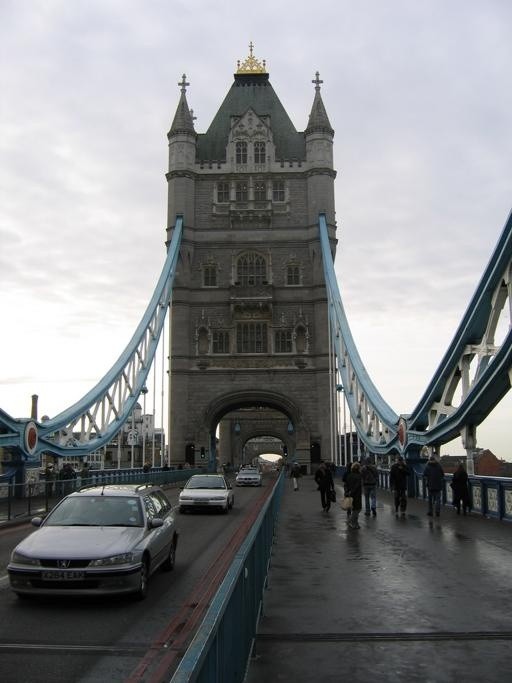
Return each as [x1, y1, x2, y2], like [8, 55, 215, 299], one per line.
[287, 420, 294, 435]
[233, 416, 241, 432]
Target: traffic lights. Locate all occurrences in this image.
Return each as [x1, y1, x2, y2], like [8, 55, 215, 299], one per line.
[201, 447, 205, 459]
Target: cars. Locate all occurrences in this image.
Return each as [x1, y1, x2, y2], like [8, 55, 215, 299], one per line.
[179, 474, 234, 513]
[235, 464, 263, 487]
[7, 483, 178, 599]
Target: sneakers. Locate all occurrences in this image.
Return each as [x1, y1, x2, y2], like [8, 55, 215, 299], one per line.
[322, 506, 468, 517]
[344, 520, 361, 530]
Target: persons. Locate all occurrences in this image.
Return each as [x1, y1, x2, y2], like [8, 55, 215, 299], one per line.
[81, 462, 89, 486]
[56, 464, 68, 497]
[388, 455, 411, 519]
[346, 461, 362, 529]
[177, 463, 184, 469]
[143, 460, 152, 482]
[341, 462, 352, 518]
[163, 463, 170, 481]
[314, 460, 334, 513]
[290, 461, 302, 492]
[423, 456, 446, 516]
[449, 463, 468, 516]
[64, 462, 77, 494]
[40, 462, 54, 498]
[360, 456, 379, 516]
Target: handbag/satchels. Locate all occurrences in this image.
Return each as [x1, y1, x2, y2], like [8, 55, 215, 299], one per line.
[330, 489, 338, 504]
[340, 496, 353, 510]
